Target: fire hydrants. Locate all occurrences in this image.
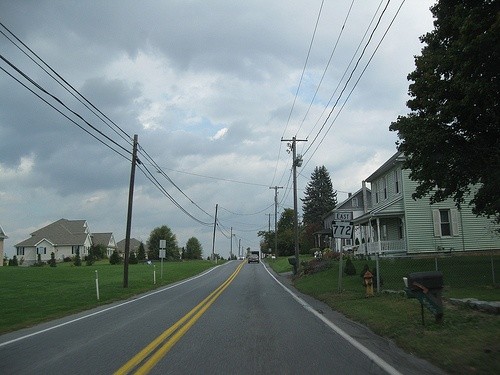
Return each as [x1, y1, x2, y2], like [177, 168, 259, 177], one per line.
[363, 269, 374, 297]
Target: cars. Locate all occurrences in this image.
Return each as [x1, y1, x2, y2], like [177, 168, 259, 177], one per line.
[248, 254, 259, 264]
